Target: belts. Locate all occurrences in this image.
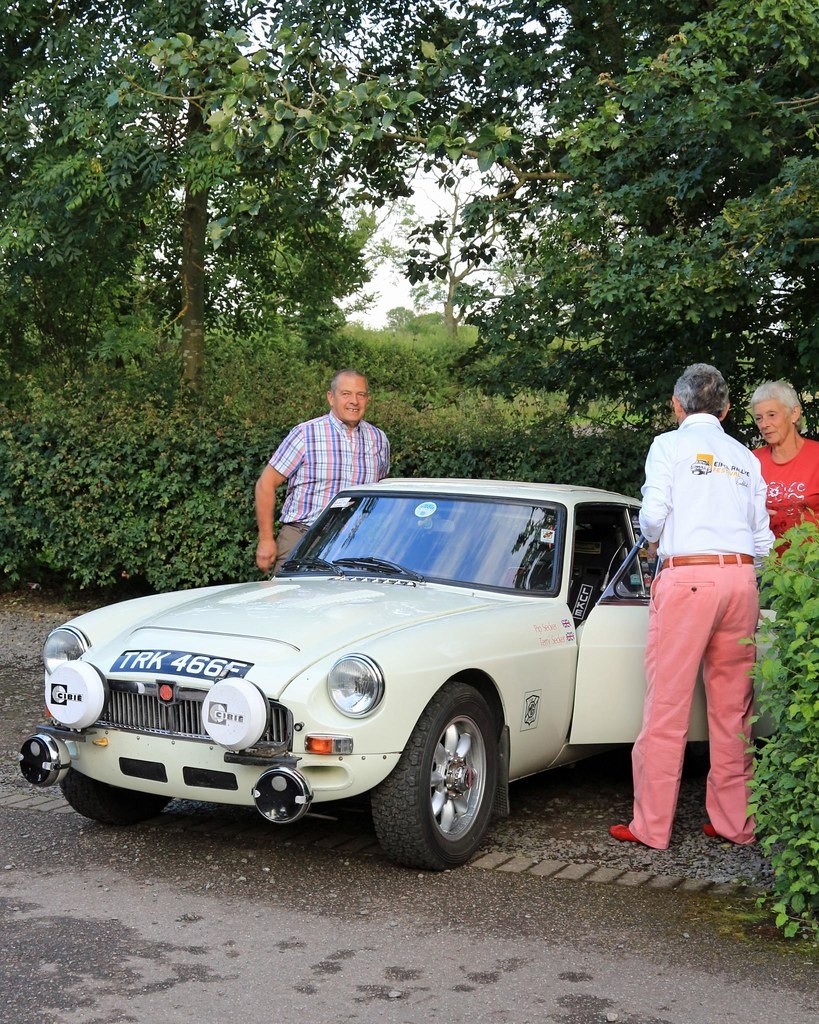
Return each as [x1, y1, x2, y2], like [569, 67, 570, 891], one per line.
[286, 522, 310, 531]
[659, 554, 754, 572]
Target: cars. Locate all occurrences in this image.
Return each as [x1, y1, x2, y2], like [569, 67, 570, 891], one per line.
[20, 477, 780, 871]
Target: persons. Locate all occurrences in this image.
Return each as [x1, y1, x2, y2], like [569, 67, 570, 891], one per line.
[255, 370, 389, 577]
[750, 381, 819, 606]
[609, 364, 775, 849]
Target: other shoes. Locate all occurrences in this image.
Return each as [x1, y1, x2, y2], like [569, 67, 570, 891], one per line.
[703, 823, 718, 837]
[610, 824, 642, 843]
